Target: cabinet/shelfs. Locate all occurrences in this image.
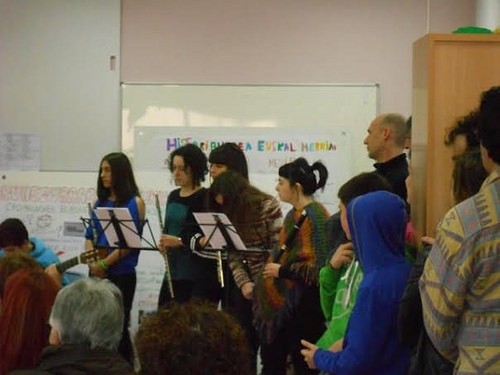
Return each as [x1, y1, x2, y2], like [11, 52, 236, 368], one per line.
[410, 34, 500, 249]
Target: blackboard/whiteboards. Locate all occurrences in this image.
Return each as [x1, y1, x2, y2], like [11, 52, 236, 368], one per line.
[119, 81, 383, 272]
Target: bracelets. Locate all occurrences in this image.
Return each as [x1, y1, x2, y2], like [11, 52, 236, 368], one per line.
[97, 259, 108, 271]
[178, 238, 182, 248]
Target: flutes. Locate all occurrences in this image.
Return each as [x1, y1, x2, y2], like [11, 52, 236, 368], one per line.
[217, 252, 225, 288]
[273, 208, 307, 264]
[154, 193, 175, 299]
[88, 202, 100, 247]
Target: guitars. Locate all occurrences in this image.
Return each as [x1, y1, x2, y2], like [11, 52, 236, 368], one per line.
[44, 246, 110, 274]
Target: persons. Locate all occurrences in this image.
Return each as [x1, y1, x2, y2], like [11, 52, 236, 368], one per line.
[85, 153, 145, 375]
[0, 217, 136, 375]
[135, 296, 254, 374]
[158, 85, 500, 375]
